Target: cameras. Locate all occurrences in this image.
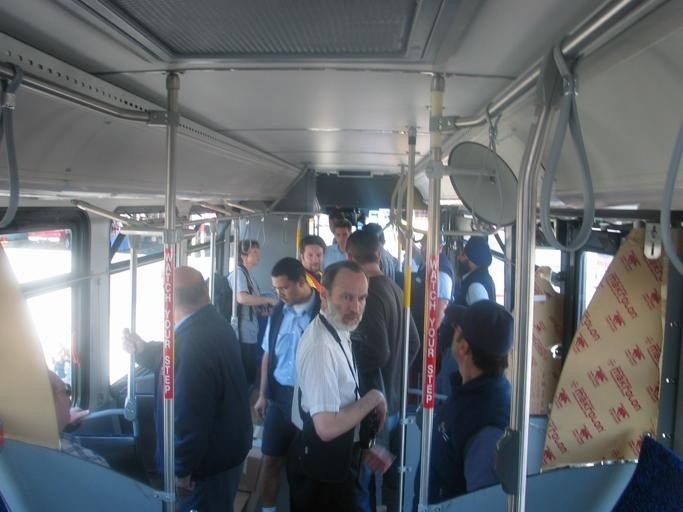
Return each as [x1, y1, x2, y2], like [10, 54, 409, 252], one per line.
[359, 414, 378, 451]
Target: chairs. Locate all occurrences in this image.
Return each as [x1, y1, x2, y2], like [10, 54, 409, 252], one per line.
[73, 375, 156, 488]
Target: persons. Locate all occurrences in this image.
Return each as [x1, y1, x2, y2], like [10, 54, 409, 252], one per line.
[47, 370, 89, 432]
[227, 218, 517, 512]
[125, 266, 252, 512]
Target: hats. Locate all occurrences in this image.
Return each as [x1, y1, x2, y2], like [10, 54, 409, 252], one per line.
[447, 300, 514, 353]
[465, 236, 491, 267]
